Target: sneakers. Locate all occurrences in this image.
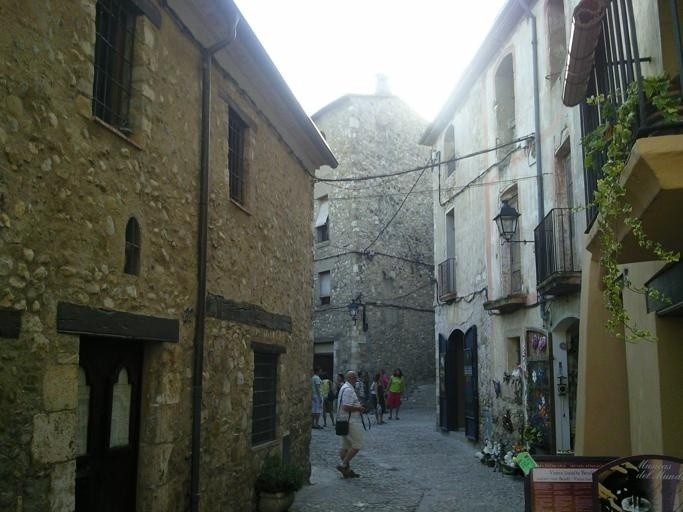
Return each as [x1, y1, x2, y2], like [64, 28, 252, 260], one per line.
[336, 464, 360, 478]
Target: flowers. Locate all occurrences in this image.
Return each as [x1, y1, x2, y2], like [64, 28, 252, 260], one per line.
[500, 357, 547, 466]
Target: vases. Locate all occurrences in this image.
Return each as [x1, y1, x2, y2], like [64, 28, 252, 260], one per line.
[500, 460, 525, 475]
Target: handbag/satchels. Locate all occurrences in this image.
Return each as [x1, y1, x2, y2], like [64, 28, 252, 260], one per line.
[336, 421, 348, 435]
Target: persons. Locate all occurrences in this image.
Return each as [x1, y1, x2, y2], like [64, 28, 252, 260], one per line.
[335, 370, 367, 479]
[312, 366, 323, 429]
[334, 373, 345, 398]
[385, 368, 404, 420]
[322, 372, 336, 427]
[355, 368, 389, 425]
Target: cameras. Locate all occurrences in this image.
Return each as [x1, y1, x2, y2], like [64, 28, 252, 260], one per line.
[362, 405, 368, 414]
